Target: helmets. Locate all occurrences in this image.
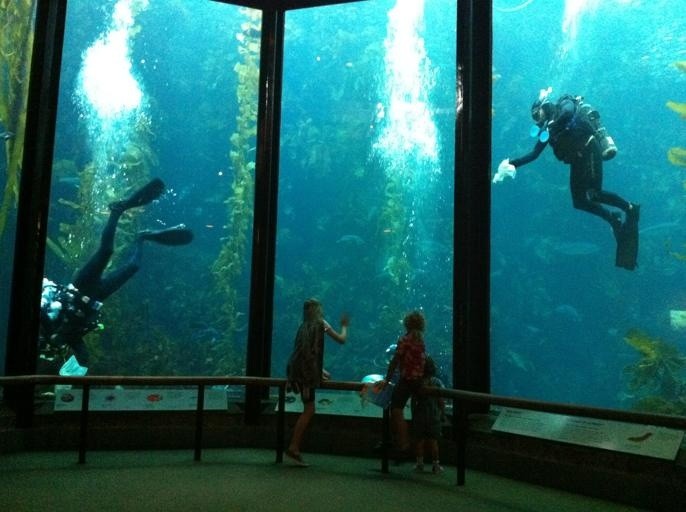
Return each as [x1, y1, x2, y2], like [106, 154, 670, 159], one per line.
[532, 98, 552, 126]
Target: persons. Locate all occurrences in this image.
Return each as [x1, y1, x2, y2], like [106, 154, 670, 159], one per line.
[285, 297, 348, 468]
[507, 93, 639, 271]
[413, 358, 446, 474]
[383, 311, 427, 451]
[40, 177, 194, 374]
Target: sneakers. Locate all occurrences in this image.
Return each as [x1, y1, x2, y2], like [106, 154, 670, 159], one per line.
[286, 451, 308, 466]
